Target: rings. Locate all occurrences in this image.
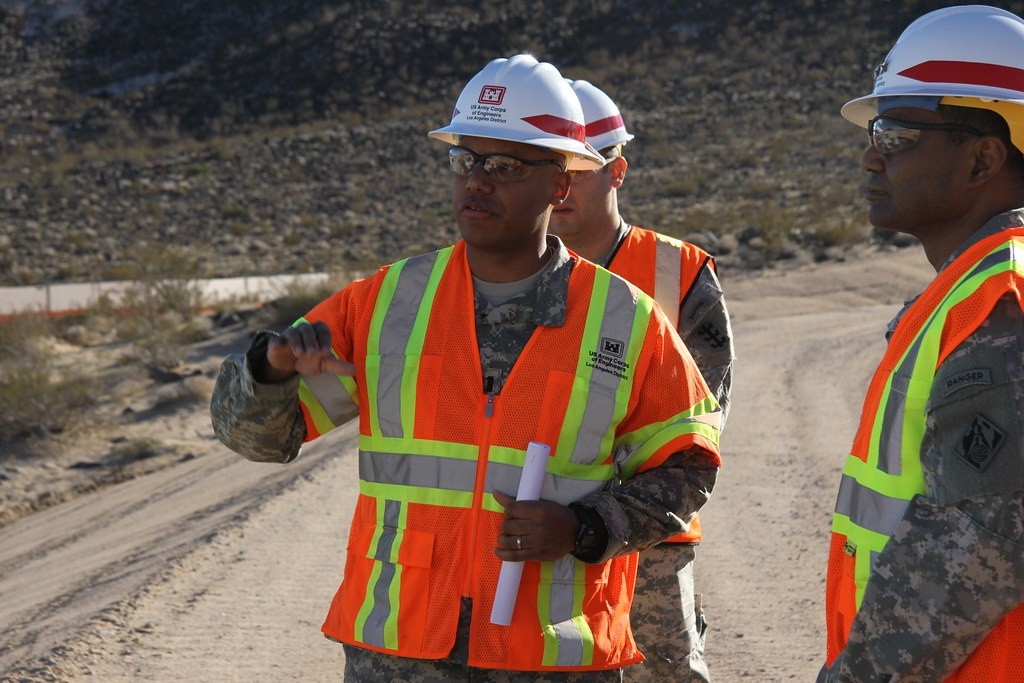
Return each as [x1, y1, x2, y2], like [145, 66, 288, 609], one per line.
[515, 536, 522, 549]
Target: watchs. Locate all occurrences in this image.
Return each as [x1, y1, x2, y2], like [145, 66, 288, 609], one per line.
[569, 499, 600, 557]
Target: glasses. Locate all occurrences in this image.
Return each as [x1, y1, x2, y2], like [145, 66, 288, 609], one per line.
[447, 144, 565, 182]
[864, 116, 989, 154]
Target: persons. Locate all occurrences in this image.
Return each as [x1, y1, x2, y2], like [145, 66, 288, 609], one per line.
[814, 6, 1023, 683]
[548, 73, 735, 683]
[208, 54, 725, 683]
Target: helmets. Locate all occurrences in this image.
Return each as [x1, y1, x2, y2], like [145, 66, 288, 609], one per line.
[564, 77, 637, 151]
[429, 55, 607, 171]
[839, 6, 1024, 162]
